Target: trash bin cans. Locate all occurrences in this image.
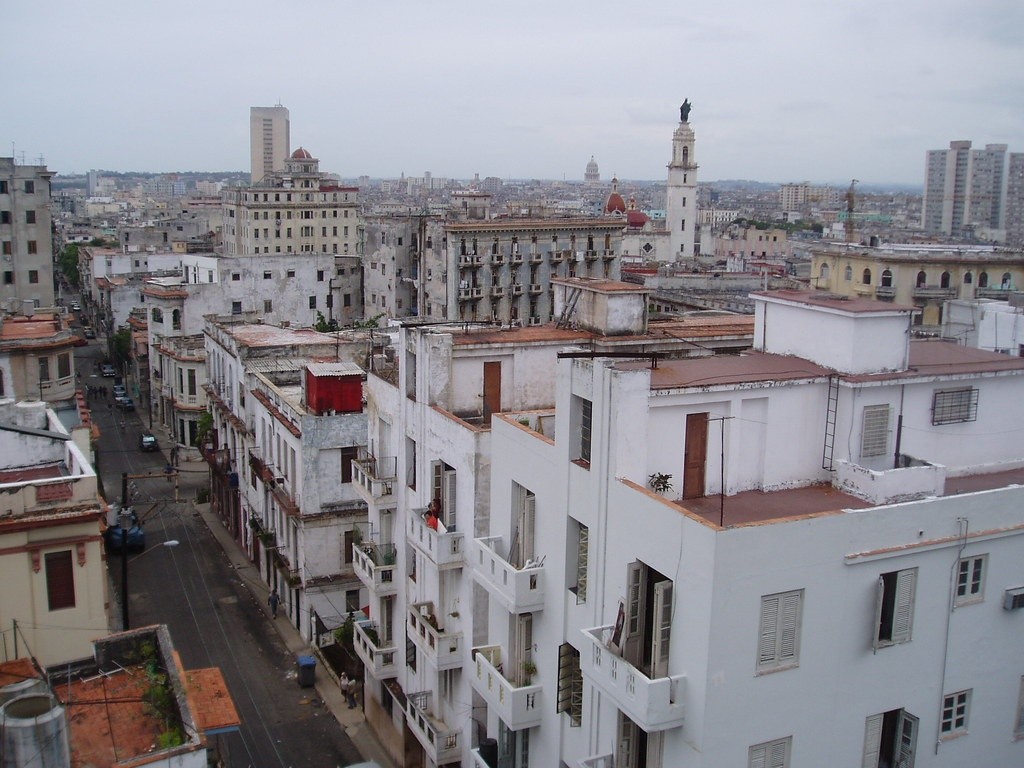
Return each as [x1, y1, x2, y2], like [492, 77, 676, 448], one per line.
[295, 655, 317, 687]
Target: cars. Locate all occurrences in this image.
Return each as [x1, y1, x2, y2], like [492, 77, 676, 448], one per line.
[85, 330, 94, 339]
[139, 434, 158, 452]
[84, 326, 92, 333]
[112, 385, 126, 396]
[98, 361, 116, 378]
[115, 396, 134, 411]
[71, 300, 89, 326]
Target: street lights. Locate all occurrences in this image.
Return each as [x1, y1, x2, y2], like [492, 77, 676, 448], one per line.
[121, 539, 180, 632]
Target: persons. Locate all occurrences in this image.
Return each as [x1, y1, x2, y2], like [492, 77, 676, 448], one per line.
[153, 368, 160, 378]
[77, 372, 81, 385]
[108, 402, 112, 415]
[421, 499, 441, 531]
[93, 360, 102, 372]
[268, 590, 281, 619]
[170, 441, 180, 467]
[165, 463, 172, 482]
[88, 385, 108, 399]
[340, 672, 357, 709]
[120, 419, 125, 433]
